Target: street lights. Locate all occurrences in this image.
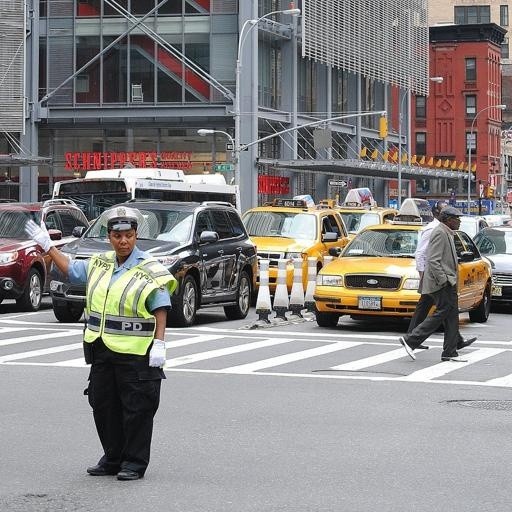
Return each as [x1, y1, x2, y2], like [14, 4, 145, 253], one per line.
[467, 102, 509, 217]
[397, 74, 444, 207]
[197, 6, 303, 214]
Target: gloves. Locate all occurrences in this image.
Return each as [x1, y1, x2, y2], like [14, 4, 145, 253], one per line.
[148, 339, 167, 369]
[25, 219, 55, 254]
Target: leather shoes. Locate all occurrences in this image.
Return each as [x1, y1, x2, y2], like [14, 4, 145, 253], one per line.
[415, 345, 430, 350]
[117, 469, 140, 481]
[457, 337, 477, 350]
[87, 461, 115, 477]
[441, 354, 468, 362]
[399, 336, 416, 361]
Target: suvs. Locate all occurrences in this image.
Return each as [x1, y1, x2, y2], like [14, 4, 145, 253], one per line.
[0, 197, 93, 310]
[45, 196, 258, 327]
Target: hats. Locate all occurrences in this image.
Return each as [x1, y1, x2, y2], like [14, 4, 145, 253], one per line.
[98, 206, 146, 231]
[438, 206, 464, 217]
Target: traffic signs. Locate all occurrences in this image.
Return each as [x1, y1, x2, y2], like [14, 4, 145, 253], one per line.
[225, 143, 248, 152]
[211, 163, 234, 172]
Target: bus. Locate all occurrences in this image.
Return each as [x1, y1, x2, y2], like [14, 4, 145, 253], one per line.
[51, 166, 241, 227]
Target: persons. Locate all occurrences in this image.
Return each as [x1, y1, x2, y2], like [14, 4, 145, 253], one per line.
[407, 201, 478, 350]
[399, 205, 468, 363]
[24, 205, 177, 481]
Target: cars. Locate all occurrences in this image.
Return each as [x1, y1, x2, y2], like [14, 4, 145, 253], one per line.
[241, 186, 512, 334]
